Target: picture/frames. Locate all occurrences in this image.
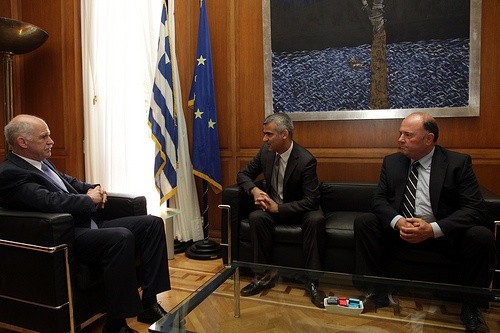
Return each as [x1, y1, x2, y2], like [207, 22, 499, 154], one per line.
[260, 0, 483, 123]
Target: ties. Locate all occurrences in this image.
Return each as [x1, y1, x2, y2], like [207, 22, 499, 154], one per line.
[401, 160, 420, 219]
[271, 154, 284, 201]
[41, 163, 70, 193]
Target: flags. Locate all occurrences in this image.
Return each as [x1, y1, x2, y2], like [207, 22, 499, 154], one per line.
[188, 0, 223, 193]
[147, 0, 178, 204]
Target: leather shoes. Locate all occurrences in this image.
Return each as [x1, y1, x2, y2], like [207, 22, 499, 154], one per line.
[357, 292, 391, 313]
[305, 279, 327, 308]
[461, 305, 489, 333]
[103, 318, 138, 333]
[240, 271, 277, 297]
[137, 303, 186, 327]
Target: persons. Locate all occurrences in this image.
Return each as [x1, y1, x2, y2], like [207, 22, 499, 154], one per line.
[236, 113, 325, 306]
[352, 112, 495, 333]
[0, 114, 185, 333]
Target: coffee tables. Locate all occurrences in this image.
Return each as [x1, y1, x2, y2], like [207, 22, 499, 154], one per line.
[148, 259, 500, 333]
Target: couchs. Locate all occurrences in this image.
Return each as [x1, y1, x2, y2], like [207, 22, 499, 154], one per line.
[0, 192, 148, 333]
[218, 178, 500, 313]
